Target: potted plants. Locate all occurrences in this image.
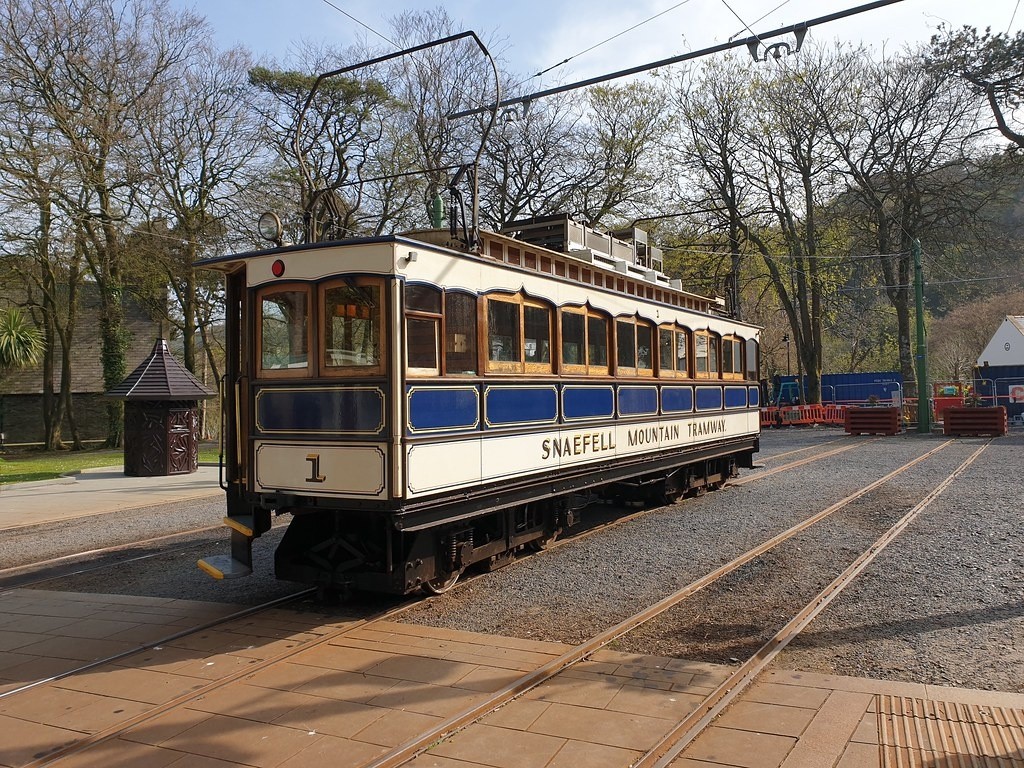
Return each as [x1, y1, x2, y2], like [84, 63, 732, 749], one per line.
[943, 392, 1007, 437]
[845, 394, 902, 436]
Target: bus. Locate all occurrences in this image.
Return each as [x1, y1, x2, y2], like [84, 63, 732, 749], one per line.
[191, 213, 763, 600]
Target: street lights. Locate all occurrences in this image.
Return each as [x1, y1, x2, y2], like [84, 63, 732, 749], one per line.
[783, 333, 790, 376]
